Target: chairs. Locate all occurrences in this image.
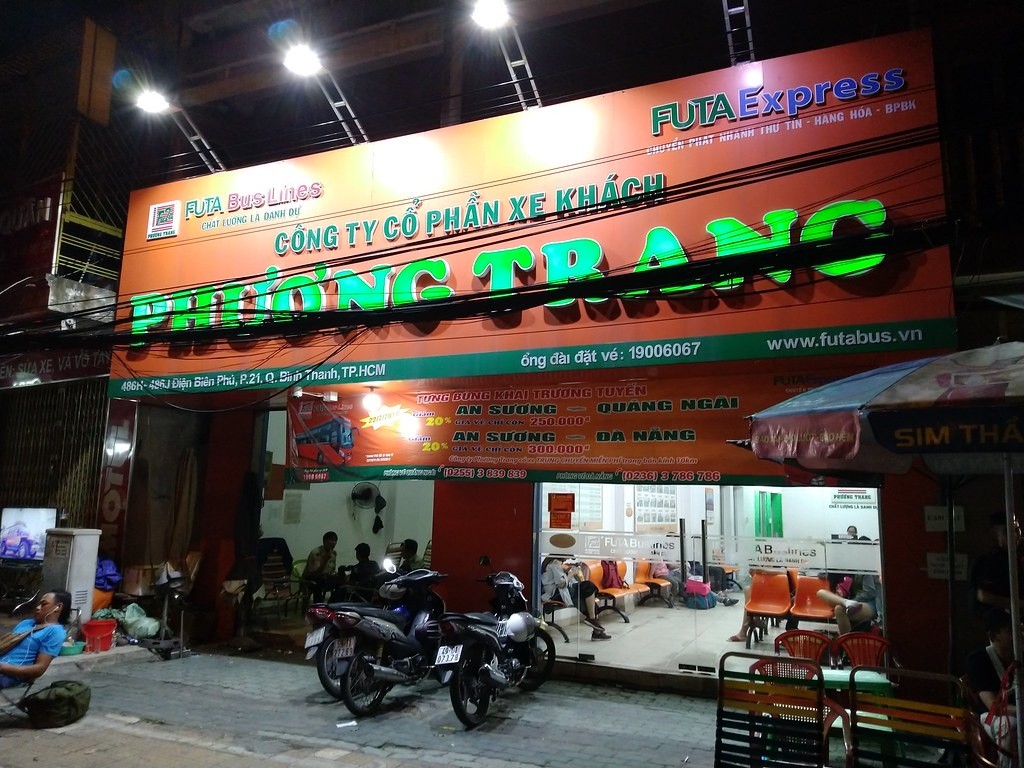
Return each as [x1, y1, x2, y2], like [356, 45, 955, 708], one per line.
[293, 556, 338, 608]
[422, 539, 432, 570]
[1, 609, 83, 727]
[253, 532, 309, 618]
[773, 629, 837, 766]
[384, 542, 402, 573]
[749, 660, 853, 768]
[837, 634, 889, 689]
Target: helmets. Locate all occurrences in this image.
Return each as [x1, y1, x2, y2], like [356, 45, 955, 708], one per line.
[506, 612, 534, 642]
[387, 581, 408, 598]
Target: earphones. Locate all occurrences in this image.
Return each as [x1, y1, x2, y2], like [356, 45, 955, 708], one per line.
[53, 606, 59, 612]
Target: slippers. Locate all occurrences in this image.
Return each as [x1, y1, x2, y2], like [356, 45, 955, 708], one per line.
[727, 636, 748, 642]
[846, 603, 863, 615]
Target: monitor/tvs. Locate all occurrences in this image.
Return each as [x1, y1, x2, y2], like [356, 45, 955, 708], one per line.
[0, 508, 58, 563]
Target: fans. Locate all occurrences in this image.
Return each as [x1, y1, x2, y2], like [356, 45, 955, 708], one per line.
[352, 481, 382, 511]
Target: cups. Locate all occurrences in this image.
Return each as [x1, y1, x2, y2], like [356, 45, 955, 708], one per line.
[59, 514, 69, 528]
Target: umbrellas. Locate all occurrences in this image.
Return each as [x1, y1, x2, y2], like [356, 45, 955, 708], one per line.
[744, 341, 1024, 767]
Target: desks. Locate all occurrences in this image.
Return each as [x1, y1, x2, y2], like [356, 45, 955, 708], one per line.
[766, 667, 906, 768]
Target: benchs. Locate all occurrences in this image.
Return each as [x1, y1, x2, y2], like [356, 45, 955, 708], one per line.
[746, 568, 883, 649]
[540, 554, 743, 644]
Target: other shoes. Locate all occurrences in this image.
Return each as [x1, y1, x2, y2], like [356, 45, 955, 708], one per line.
[679, 598, 686, 603]
[723, 597, 739, 606]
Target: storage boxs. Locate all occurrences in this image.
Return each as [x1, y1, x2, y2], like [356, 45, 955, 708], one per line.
[124, 564, 166, 597]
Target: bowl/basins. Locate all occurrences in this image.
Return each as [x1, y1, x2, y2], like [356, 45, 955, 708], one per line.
[59, 641, 88, 656]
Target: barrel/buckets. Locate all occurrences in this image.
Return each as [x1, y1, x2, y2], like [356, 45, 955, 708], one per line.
[81, 619, 118, 652]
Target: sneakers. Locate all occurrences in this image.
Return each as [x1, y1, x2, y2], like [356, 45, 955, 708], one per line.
[583, 617, 605, 630]
[591, 630, 611, 641]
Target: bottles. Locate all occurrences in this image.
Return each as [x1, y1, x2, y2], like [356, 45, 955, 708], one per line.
[327, 560, 335, 576]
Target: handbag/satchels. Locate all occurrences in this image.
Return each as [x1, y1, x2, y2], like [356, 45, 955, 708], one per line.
[684, 575, 716, 609]
[16, 679, 91, 729]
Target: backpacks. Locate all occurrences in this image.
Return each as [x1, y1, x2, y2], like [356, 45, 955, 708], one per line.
[600, 560, 624, 589]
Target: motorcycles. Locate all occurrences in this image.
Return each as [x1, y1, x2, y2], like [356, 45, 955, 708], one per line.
[303, 557, 455, 718]
[433, 554, 557, 727]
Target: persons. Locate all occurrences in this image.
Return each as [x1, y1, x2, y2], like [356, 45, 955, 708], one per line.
[396, 539, 428, 576]
[847, 525, 880, 544]
[349, 543, 380, 584]
[0, 591, 72, 690]
[817, 569, 883, 635]
[650, 532, 740, 609]
[727, 540, 797, 642]
[974, 611, 1018, 715]
[541, 551, 611, 641]
[297, 531, 338, 599]
